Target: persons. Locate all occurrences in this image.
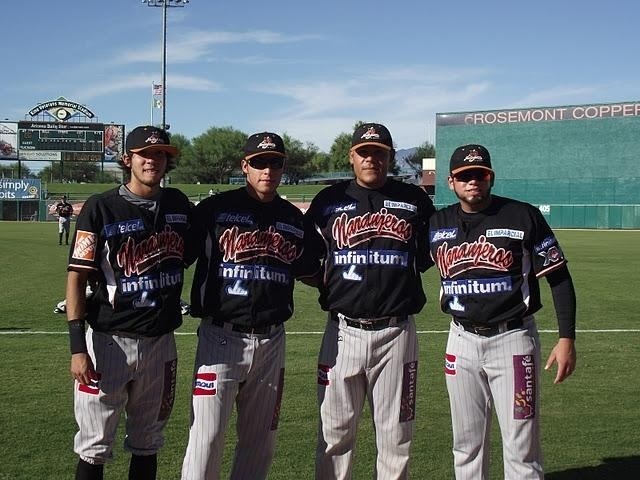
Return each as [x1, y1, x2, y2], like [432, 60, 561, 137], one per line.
[181, 131, 321, 479]
[428, 143, 578, 480]
[64, 124, 196, 480]
[303, 123, 439, 480]
[104, 125, 120, 161]
[55, 195, 74, 246]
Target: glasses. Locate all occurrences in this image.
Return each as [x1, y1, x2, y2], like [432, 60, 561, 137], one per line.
[246, 156, 283, 170]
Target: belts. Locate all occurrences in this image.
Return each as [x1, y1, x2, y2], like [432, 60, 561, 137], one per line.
[345, 317, 407, 331]
[213, 319, 272, 334]
[454, 321, 522, 336]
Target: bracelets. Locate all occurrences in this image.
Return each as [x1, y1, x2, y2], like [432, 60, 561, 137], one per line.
[66, 318, 89, 356]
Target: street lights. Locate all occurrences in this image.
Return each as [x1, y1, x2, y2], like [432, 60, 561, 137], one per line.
[139, 0, 189, 189]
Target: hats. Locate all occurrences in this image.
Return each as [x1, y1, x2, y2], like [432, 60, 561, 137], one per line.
[449, 145, 493, 176]
[350, 124, 394, 152]
[244, 131, 284, 159]
[125, 126, 177, 158]
